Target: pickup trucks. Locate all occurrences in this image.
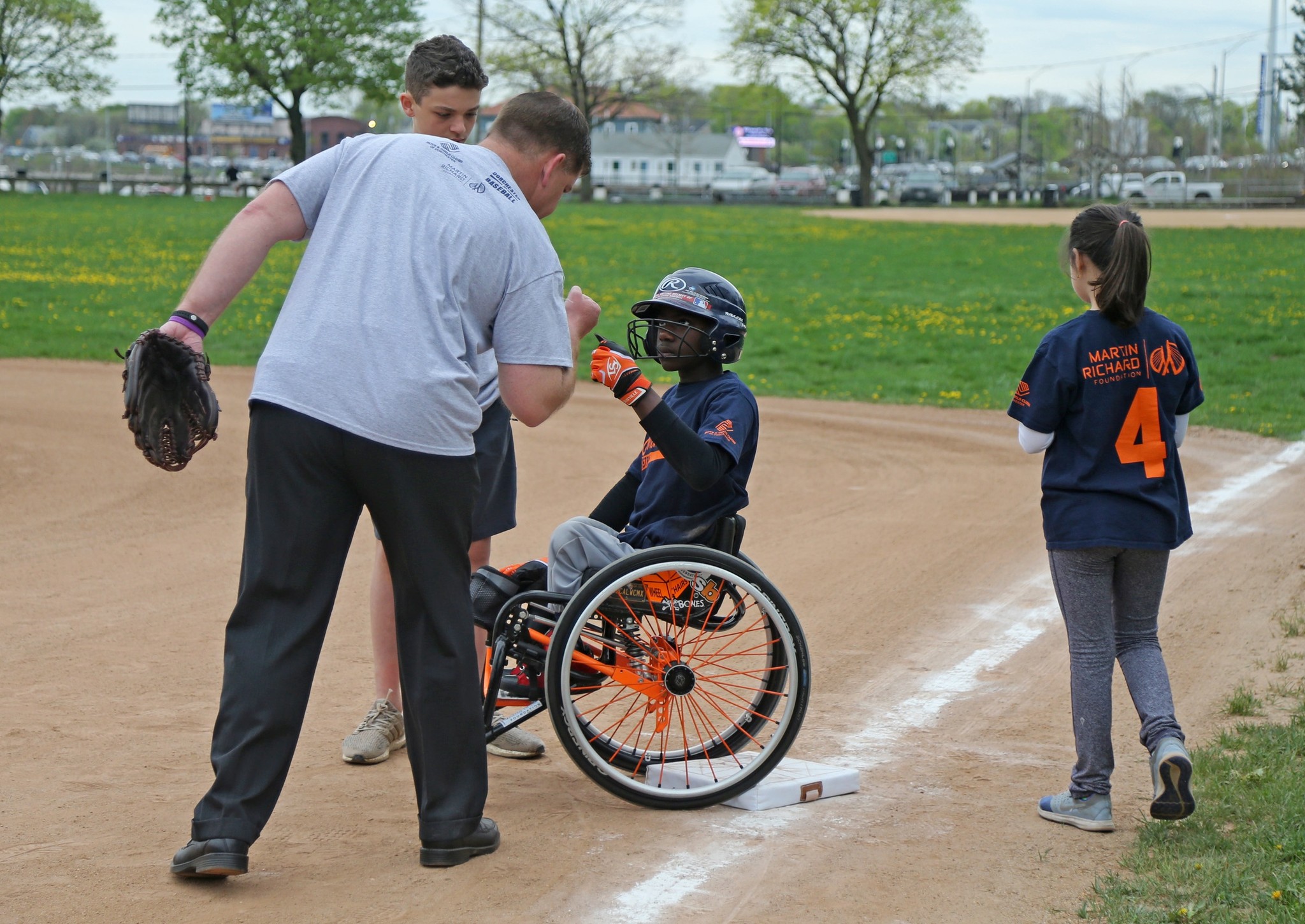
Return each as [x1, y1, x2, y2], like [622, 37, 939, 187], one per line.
[1121, 170, 1224, 208]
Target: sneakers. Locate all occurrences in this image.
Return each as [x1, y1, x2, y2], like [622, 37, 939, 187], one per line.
[341, 690, 405, 761]
[500, 628, 599, 697]
[1149, 737, 1196, 820]
[1038, 794, 1113, 831]
[485, 712, 546, 757]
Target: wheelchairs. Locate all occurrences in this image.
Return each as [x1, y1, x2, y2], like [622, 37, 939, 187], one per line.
[480, 513, 811, 810]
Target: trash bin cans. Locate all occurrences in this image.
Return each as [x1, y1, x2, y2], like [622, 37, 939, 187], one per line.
[1044, 184, 1059, 207]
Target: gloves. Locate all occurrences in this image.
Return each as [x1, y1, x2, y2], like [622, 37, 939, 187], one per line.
[591, 334, 651, 407]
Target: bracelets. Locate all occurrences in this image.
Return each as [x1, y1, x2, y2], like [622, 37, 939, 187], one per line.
[171, 311, 209, 336]
[168, 316, 205, 340]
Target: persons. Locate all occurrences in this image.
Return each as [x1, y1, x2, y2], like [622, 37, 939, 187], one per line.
[119, 93, 596, 873]
[339, 33, 547, 765]
[500, 267, 759, 697]
[1005, 203, 1196, 833]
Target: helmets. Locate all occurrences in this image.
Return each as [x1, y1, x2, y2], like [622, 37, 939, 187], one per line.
[630, 268, 747, 363]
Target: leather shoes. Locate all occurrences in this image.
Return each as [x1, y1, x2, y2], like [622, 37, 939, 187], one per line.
[170, 839, 247, 875]
[419, 818, 500, 866]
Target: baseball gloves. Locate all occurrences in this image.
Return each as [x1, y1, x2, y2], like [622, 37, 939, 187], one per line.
[123, 328, 219, 473]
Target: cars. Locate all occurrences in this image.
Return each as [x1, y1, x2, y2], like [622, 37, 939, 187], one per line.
[707, 153, 1144, 202]
